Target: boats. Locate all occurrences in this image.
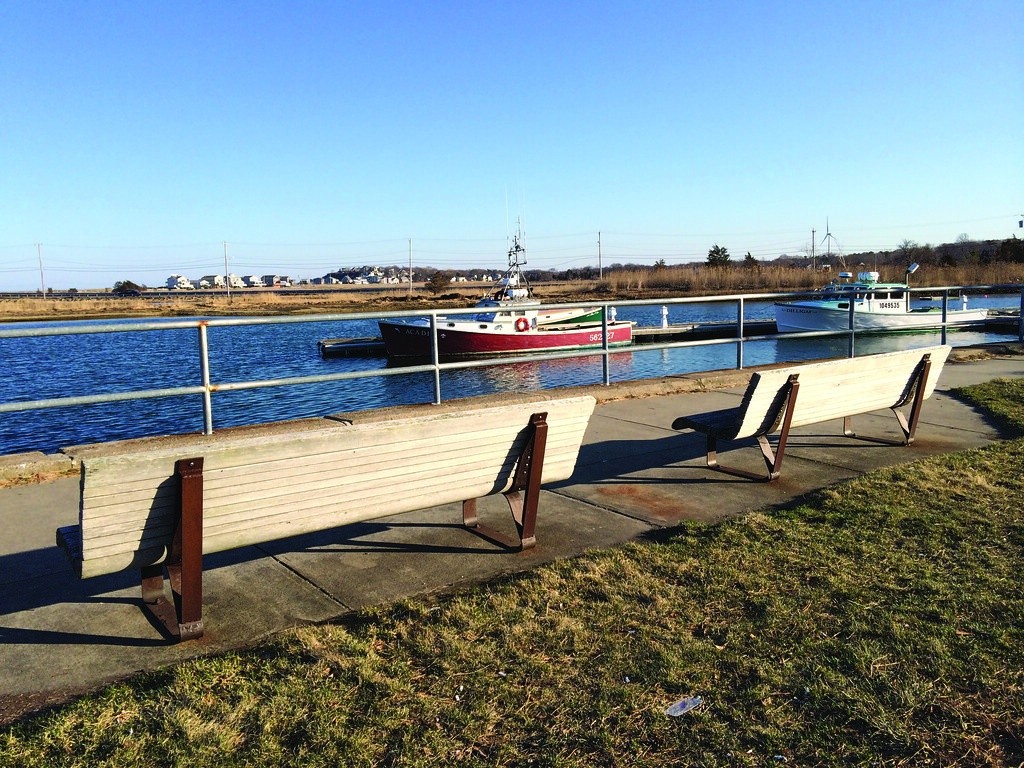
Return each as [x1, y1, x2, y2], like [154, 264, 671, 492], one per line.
[772, 251, 1021, 339]
[377, 181, 639, 364]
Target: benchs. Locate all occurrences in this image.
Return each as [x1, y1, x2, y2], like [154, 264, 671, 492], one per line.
[57, 394, 596, 638]
[672, 345, 953, 482]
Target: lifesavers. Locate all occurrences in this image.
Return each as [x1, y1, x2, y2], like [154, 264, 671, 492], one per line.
[515, 317, 529, 332]
